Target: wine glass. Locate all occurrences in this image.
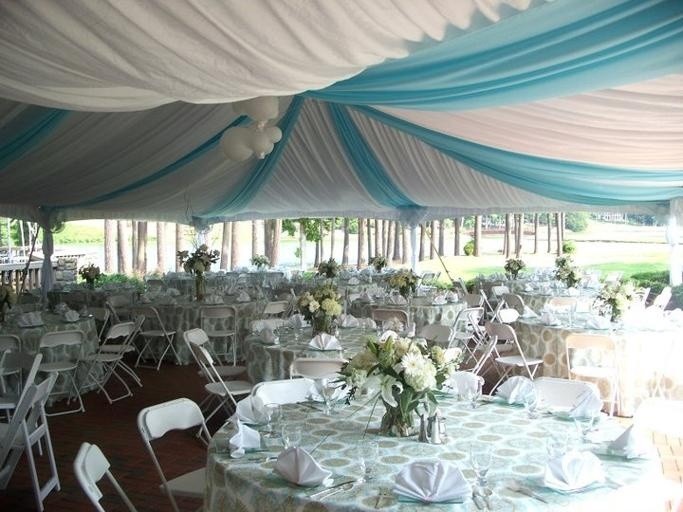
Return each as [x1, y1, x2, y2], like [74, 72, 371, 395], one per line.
[469, 440, 495, 501]
[262, 402, 282, 439]
[355, 440, 378, 487]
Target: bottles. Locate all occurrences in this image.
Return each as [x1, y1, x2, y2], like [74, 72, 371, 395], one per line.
[418, 412, 441, 445]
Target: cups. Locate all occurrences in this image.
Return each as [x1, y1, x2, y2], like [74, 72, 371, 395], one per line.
[282, 424, 301, 450]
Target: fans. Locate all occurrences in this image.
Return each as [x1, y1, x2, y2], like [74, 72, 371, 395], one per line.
[193, 216, 213, 234]
[37, 202, 67, 233]
[399, 208, 418, 230]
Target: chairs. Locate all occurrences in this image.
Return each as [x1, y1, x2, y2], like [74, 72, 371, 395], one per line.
[251, 378, 318, 412]
[105, 270, 488, 379]
[0, 348, 61, 512]
[470, 271, 683, 421]
[137, 398, 212, 512]
[184, 327, 248, 412]
[190, 343, 254, 420]
[73, 441, 136, 511]
[2, 272, 142, 416]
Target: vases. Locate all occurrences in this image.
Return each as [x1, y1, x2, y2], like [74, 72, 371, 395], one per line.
[380, 406, 419, 437]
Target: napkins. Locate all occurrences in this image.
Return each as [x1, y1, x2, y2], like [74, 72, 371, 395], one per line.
[393, 458, 473, 504]
[274, 445, 336, 486]
[228, 418, 262, 457]
[235, 394, 274, 423]
[449, 371, 484, 396]
[543, 451, 605, 490]
[608, 422, 645, 453]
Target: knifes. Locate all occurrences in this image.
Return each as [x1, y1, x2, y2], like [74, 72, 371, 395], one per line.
[308, 480, 354, 496]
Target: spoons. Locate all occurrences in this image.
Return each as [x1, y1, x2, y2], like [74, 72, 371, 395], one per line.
[318, 482, 353, 501]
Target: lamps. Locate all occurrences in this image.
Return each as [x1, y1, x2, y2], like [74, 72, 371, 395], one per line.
[223, 96, 282, 162]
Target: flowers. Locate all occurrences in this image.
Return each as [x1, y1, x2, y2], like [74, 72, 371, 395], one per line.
[317, 256, 344, 277]
[175, 244, 220, 273]
[339, 330, 462, 404]
[368, 255, 389, 269]
[78, 264, 100, 286]
[250, 254, 271, 270]
[504, 259, 525, 277]
[554, 254, 577, 287]
